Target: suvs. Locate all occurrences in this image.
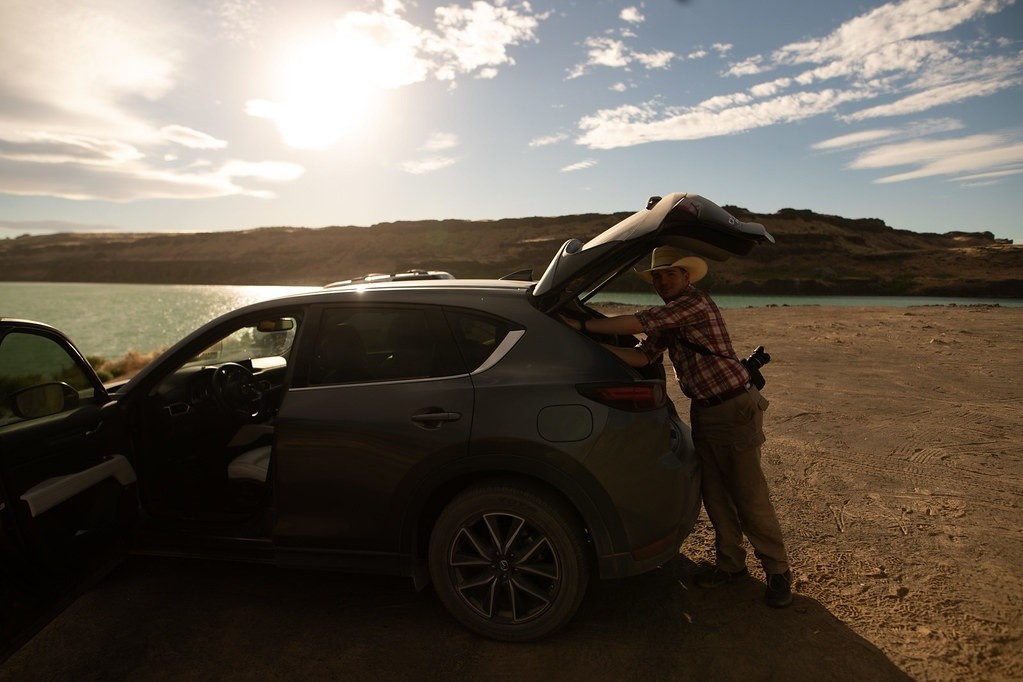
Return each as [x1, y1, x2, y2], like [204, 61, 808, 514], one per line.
[0, 190, 780, 667]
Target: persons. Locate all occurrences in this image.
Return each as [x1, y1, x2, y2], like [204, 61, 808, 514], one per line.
[557, 244, 793, 606]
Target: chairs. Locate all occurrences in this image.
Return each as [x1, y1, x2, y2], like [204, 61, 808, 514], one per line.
[317, 324, 373, 384]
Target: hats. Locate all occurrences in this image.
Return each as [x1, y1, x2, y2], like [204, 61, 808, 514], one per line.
[634, 245, 708, 284]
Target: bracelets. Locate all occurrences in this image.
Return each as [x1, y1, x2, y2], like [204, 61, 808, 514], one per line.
[578, 319, 586, 331]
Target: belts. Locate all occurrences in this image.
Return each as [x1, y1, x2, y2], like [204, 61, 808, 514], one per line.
[695, 378, 752, 408]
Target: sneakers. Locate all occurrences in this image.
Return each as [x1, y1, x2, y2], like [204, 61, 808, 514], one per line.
[696, 565, 749, 588]
[765, 568, 792, 608]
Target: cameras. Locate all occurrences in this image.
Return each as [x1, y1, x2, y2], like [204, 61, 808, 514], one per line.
[739, 345, 771, 391]
